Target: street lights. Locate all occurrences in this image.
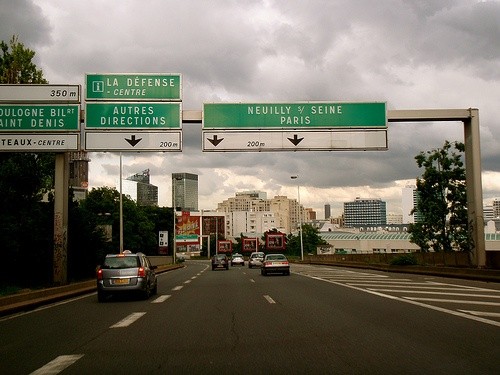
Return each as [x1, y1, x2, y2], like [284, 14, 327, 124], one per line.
[290, 175, 304, 261]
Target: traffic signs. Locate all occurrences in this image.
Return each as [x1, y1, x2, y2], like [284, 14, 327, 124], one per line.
[1, 71, 389, 153]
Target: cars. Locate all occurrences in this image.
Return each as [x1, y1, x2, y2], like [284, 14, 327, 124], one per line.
[231, 253, 244, 266]
[212, 254, 229, 271]
[260, 254, 290, 276]
[249, 252, 265, 269]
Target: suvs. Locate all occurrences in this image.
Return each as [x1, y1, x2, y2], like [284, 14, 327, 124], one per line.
[96, 249, 158, 299]
